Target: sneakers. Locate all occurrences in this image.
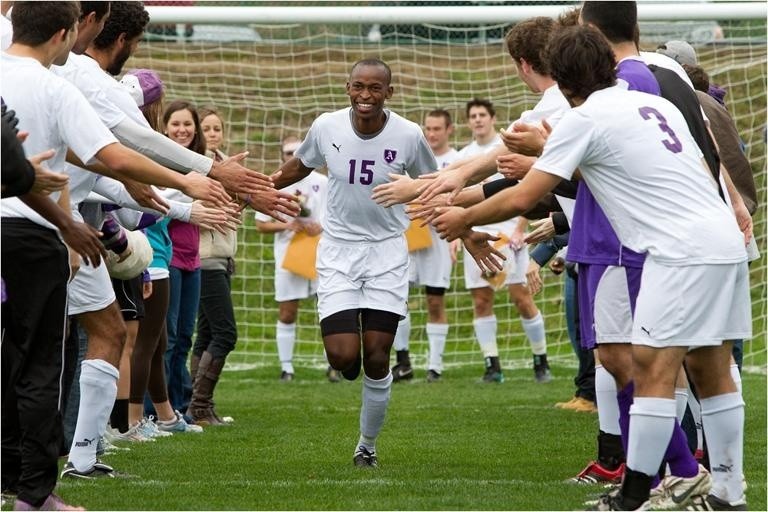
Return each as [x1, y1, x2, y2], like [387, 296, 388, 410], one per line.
[14, 492, 85, 512]
[425, 370, 439, 380]
[98, 410, 204, 457]
[280, 372, 293, 381]
[60, 460, 136, 479]
[480, 370, 504, 383]
[392, 365, 413, 381]
[555, 396, 595, 413]
[326, 366, 341, 382]
[352, 447, 378, 467]
[535, 369, 551, 381]
[565, 460, 750, 512]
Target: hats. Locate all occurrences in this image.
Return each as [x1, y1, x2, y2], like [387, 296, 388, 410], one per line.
[654, 41, 697, 68]
[120, 69, 164, 106]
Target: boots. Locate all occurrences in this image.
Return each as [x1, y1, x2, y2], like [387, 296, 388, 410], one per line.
[187, 352, 227, 425]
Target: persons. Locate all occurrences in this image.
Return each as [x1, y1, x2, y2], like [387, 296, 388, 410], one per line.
[233, 60, 507, 468]
[3, 2, 303, 509]
[255, 135, 344, 382]
[367, 2, 759, 511]
